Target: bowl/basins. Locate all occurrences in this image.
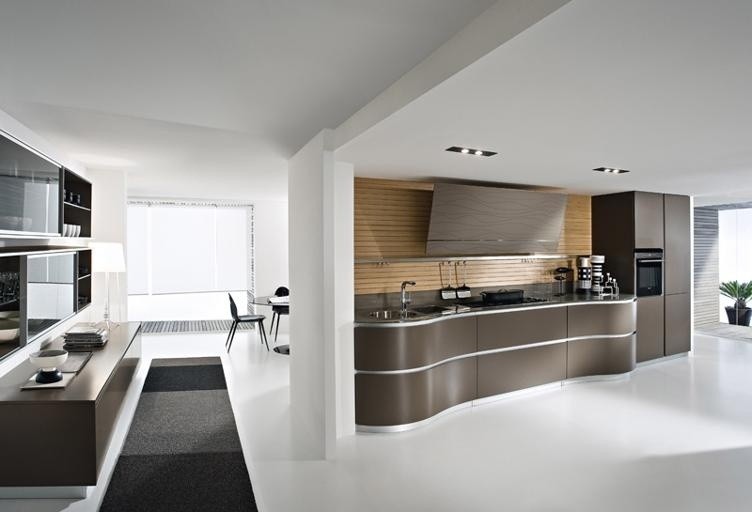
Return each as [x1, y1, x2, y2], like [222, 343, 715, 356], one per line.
[0, 320, 20, 341]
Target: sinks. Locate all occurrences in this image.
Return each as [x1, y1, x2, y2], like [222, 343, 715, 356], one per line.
[368, 310, 423, 319]
[410, 304, 456, 316]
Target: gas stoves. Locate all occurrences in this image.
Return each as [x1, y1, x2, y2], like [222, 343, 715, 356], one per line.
[455, 297, 550, 308]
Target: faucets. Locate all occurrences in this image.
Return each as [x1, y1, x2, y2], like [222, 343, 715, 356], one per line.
[400, 280, 416, 309]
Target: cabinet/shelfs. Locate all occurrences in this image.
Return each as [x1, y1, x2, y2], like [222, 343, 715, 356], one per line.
[566, 287, 637, 386]
[590, 191, 691, 368]
[474, 289, 568, 406]
[0, 321, 143, 501]
[356, 298, 477, 432]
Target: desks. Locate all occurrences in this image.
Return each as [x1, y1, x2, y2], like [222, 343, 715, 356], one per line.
[250, 295, 289, 355]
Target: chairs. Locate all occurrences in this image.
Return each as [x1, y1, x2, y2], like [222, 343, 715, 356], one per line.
[225, 293, 270, 354]
[269, 286, 288, 341]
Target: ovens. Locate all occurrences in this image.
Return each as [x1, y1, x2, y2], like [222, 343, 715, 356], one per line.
[634, 252, 664, 298]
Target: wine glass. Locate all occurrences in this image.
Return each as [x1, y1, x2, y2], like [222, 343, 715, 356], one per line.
[28, 350, 68, 383]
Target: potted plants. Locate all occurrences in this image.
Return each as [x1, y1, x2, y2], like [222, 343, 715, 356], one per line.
[718, 280, 752, 327]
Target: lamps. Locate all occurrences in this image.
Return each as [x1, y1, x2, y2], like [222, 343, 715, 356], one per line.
[89, 241, 126, 333]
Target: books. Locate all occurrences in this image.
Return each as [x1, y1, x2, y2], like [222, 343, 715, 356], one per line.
[64, 322, 110, 350]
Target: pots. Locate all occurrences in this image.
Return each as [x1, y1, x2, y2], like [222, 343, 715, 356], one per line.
[479, 288, 524, 302]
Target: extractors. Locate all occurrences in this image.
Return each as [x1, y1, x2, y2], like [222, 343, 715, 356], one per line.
[424, 183, 569, 254]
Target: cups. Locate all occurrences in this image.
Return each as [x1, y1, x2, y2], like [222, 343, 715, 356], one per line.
[63, 223, 82, 237]
